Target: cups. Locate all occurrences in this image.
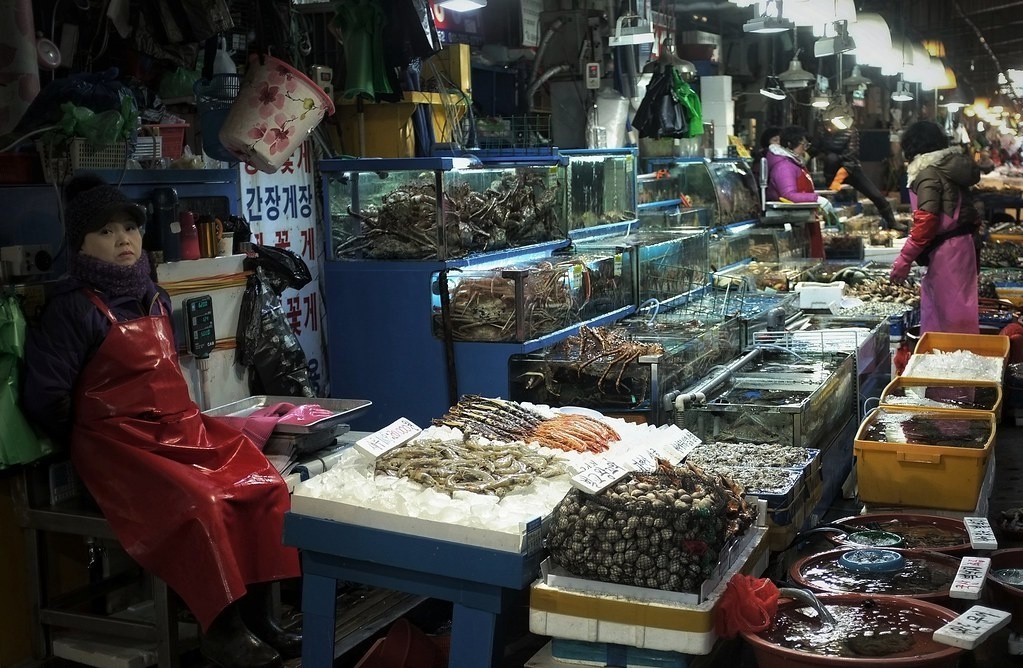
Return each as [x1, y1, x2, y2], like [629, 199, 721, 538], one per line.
[216, 232, 234, 256]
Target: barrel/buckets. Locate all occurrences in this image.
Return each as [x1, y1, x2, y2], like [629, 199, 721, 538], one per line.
[219, 57, 336, 175]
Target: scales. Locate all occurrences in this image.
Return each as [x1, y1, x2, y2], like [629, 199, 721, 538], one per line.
[758, 156, 812, 218]
[181, 294, 352, 457]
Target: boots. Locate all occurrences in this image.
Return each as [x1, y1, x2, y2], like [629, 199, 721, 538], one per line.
[879, 201, 908, 231]
[197, 603, 280, 668]
[239, 581, 303, 658]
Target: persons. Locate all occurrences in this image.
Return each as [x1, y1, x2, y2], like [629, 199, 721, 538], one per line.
[968, 137, 1023, 174]
[749, 125, 834, 215]
[890, 121, 984, 334]
[14, 171, 303, 668]
[806, 108, 908, 231]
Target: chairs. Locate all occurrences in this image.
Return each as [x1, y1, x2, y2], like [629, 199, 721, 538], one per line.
[10, 464, 282, 668]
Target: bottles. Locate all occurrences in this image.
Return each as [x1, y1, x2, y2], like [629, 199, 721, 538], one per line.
[179, 212, 201, 260]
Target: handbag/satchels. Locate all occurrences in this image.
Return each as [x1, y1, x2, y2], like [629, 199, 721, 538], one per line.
[914, 223, 972, 266]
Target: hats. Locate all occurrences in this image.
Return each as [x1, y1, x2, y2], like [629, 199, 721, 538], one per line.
[64, 174, 147, 259]
[760, 126, 781, 144]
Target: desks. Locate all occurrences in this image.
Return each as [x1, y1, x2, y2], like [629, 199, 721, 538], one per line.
[283, 513, 548, 667]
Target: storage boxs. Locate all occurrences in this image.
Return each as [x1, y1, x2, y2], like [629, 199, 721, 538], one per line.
[311, 74, 1010, 668]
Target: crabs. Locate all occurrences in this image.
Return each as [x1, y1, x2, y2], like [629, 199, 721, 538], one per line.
[334, 177, 560, 259]
[435, 259, 665, 407]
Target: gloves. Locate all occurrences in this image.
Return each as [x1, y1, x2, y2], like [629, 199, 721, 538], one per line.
[889, 237, 923, 285]
[211, 402, 334, 451]
[828, 167, 849, 192]
[817, 195, 834, 214]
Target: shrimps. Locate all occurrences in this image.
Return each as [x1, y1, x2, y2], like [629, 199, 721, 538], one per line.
[377, 436, 569, 498]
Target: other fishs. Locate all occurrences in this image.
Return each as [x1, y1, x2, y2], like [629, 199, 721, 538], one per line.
[846, 277, 921, 307]
[686, 440, 812, 491]
[440, 393, 621, 454]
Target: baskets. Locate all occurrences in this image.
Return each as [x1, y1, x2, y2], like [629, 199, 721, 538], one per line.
[132, 135, 163, 161]
[138, 123, 190, 160]
[34, 135, 128, 184]
[193, 73, 246, 163]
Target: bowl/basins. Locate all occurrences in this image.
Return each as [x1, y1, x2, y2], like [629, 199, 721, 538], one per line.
[741, 512, 1023, 668]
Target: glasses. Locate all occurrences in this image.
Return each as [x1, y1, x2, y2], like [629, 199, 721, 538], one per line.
[795, 141, 811, 150]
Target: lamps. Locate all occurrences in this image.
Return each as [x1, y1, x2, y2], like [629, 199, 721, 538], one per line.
[435, 0, 487, 12]
[607, 0, 655, 46]
[742, 0, 976, 133]
[641, 0, 698, 72]
